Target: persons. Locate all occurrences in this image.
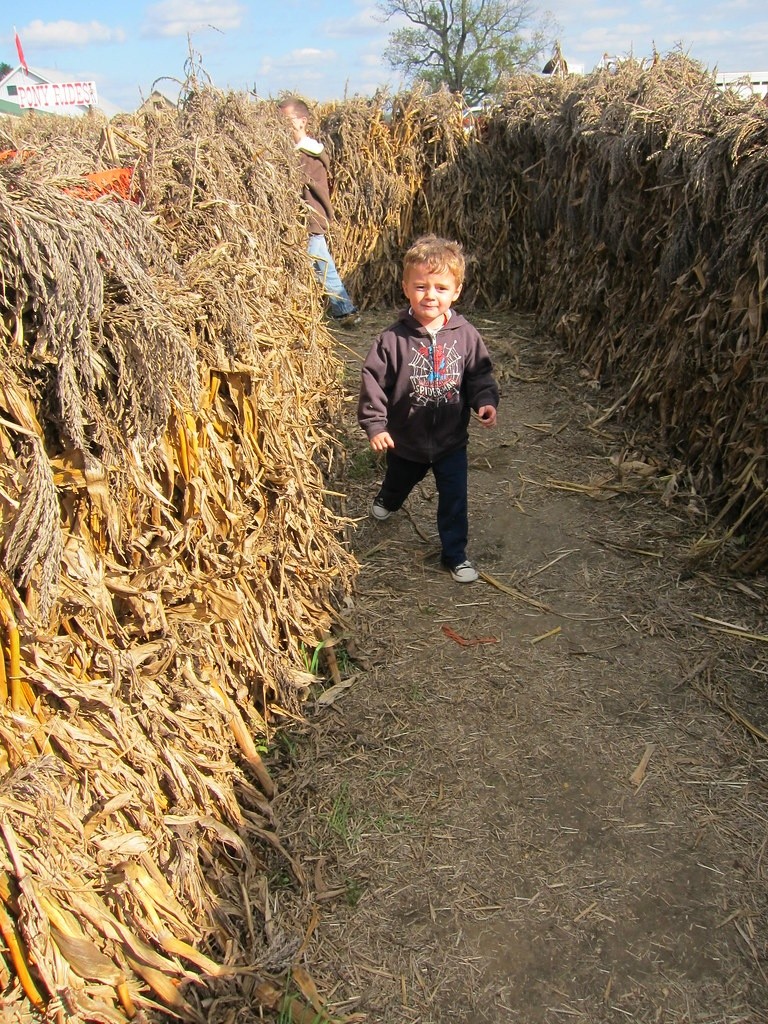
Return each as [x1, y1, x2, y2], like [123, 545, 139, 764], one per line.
[358, 234, 501, 584]
[276, 98, 362, 328]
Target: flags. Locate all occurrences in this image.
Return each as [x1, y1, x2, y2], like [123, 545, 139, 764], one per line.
[14, 26, 29, 75]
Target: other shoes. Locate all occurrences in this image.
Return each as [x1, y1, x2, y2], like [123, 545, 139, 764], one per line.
[340, 313, 362, 325]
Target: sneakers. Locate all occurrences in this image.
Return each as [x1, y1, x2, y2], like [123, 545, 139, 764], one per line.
[450, 560, 478, 582]
[371, 491, 392, 520]
